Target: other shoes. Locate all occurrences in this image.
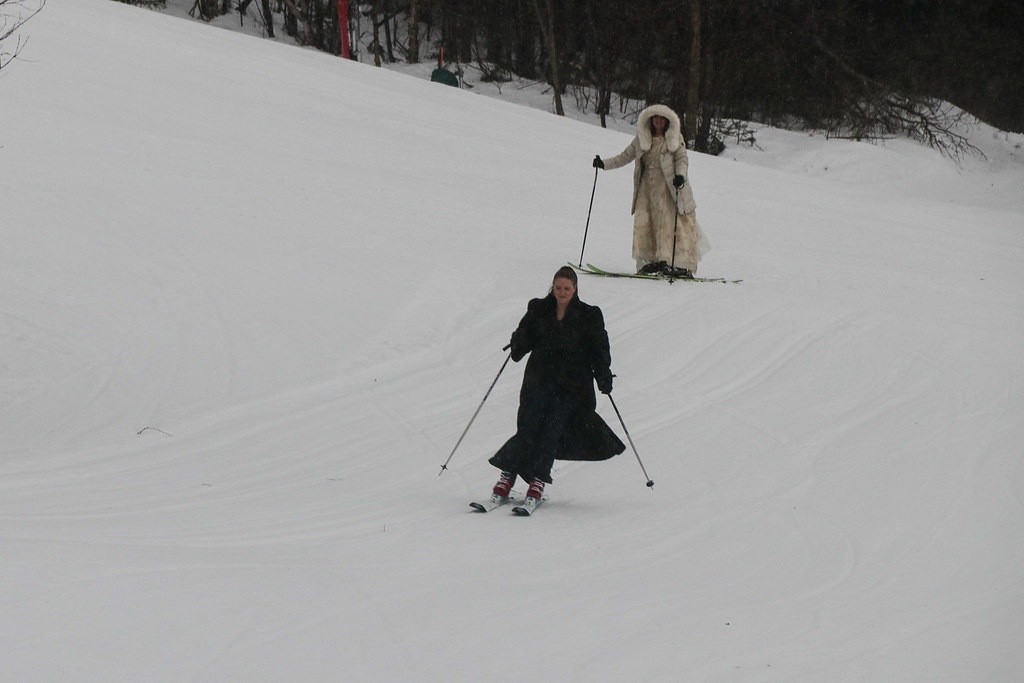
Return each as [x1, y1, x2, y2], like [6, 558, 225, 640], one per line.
[642, 262, 690, 277]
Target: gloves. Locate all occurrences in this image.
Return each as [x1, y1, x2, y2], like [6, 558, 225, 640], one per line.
[672, 176, 684, 186]
[597, 372, 613, 394]
[511, 327, 526, 348]
[593, 155, 604, 169]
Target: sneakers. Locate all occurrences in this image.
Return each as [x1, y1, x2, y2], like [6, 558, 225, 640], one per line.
[527, 477, 544, 500]
[493, 472, 517, 498]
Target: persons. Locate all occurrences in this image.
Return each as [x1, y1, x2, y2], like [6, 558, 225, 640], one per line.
[488, 267, 625, 507]
[593, 103, 711, 278]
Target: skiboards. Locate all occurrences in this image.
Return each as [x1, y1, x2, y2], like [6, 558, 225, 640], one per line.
[566, 261, 745, 284]
[468, 492, 550, 516]
